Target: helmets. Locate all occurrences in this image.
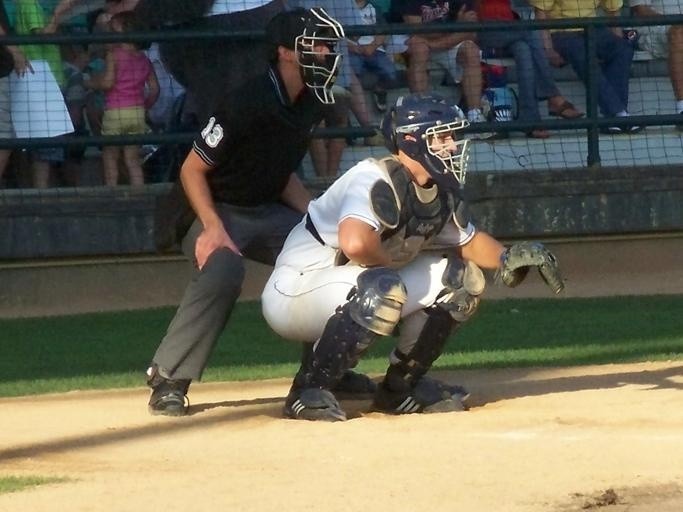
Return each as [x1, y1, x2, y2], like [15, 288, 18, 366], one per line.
[378, 93, 473, 190]
[275, 6, 346, 105]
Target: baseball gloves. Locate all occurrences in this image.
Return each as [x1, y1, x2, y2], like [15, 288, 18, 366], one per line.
[500, 240, 564, 295]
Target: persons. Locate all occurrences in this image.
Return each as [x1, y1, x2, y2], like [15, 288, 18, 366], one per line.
[145, 10, 348, 417]
[259, 93, 566, 424]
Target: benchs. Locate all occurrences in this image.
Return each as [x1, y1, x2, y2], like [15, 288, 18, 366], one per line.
[300, 50, 682, 178]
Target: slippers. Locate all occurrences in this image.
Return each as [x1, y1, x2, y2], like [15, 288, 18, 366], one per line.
[524, 98, 586, 139]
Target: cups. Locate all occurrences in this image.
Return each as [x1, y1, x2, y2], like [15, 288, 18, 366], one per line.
[625, 29, 637, 48]
[358, 34, 374, 47]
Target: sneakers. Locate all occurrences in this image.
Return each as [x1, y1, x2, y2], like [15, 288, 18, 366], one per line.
[148, 373, 192, 415]
[464, 107, 496, 140]
[291, 365, 377, 397]
[599, 123, 643, 136]
[281, 384, 347, 423]
[371, 380, 469, 413]
